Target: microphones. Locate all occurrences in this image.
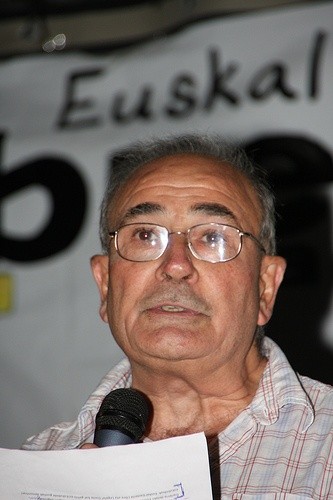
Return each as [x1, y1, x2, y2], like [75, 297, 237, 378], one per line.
[94, 388, 153, 448]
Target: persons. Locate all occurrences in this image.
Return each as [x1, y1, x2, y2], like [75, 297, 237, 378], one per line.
[18, 136, 333, 500]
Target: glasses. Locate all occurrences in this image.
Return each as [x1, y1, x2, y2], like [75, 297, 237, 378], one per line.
[106, 223, 266, 262]
